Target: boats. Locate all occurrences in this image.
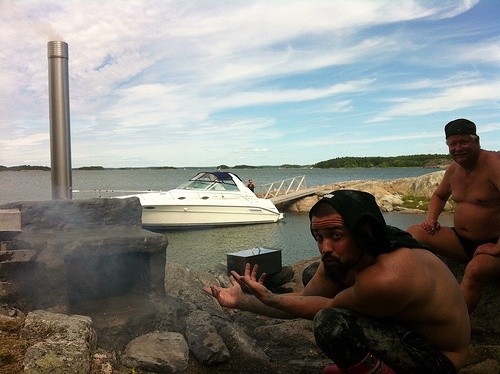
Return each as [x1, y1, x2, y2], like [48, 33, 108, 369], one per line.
[107, 171, 284, 229]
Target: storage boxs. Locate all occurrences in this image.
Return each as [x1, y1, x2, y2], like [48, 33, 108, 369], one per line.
[227, 247, 282, 280]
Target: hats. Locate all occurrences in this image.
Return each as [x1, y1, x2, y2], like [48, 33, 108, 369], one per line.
[445, 119, 476, 139]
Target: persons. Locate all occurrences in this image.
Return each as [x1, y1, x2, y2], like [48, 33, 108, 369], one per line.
[406, 118, 500, 311]
[202, 189, 471, 373]
[246, 179, 256, 193]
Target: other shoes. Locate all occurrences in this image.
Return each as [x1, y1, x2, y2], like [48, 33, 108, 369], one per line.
[323, 352, 396, 374]
